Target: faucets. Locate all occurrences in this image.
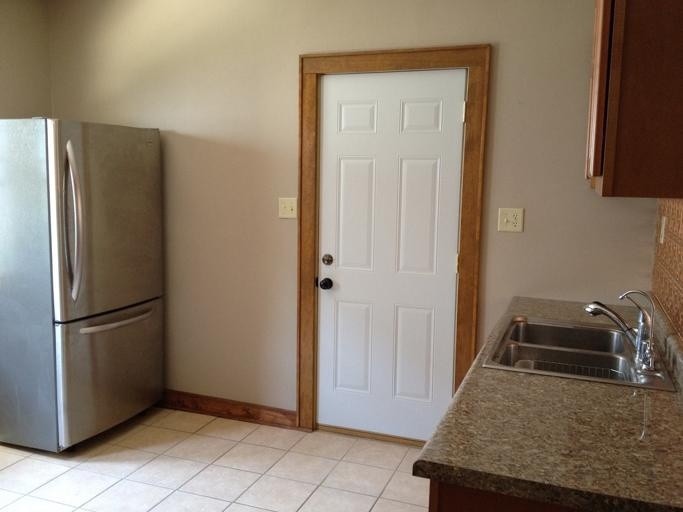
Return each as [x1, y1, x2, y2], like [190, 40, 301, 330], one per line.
[585, 299, 634, 346]
[619, 288, 657, 350]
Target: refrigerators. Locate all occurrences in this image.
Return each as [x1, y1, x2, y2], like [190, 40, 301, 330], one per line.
[1, 115, 166, 455]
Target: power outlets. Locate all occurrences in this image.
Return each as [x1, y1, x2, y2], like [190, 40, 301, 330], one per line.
[496, 206, 525, 234]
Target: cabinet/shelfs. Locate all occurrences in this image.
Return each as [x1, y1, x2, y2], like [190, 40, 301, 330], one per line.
[580, 0, 683, 199]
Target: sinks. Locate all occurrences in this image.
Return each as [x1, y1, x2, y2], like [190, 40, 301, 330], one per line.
[485, 341, 653, 387]
[509, 312, 639, 360]
